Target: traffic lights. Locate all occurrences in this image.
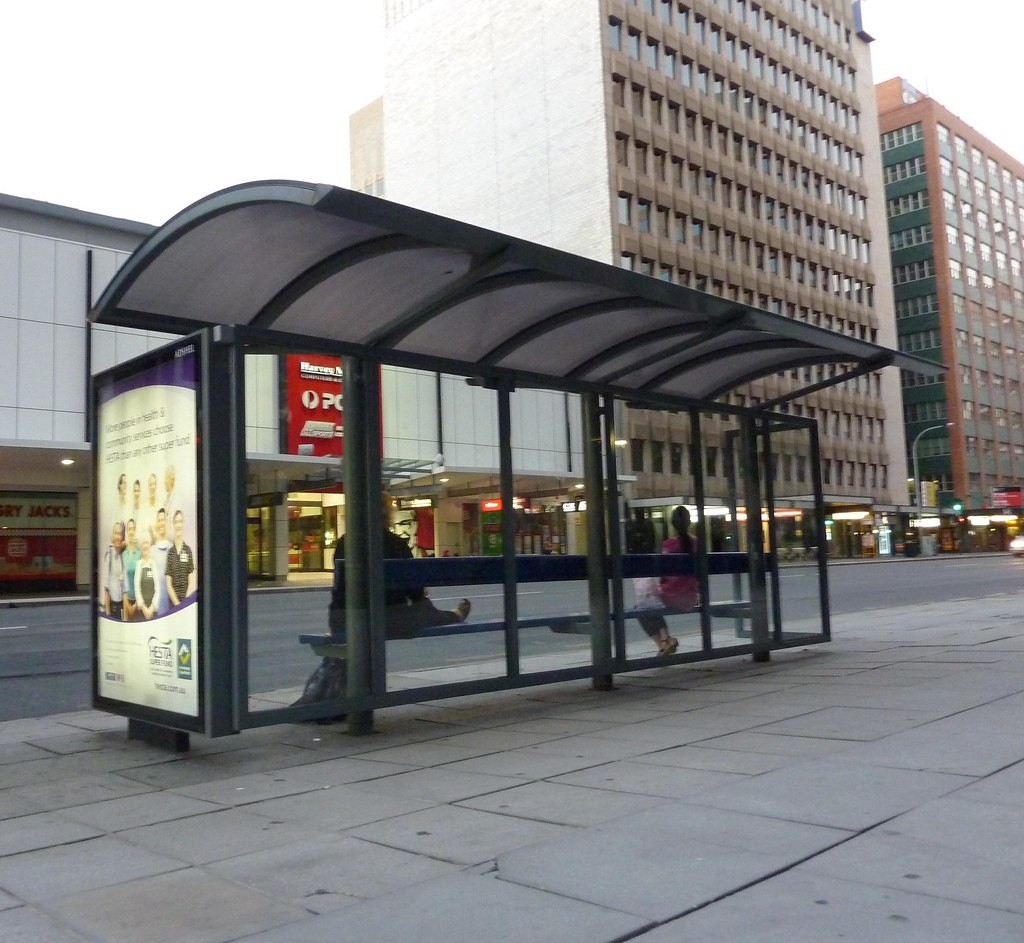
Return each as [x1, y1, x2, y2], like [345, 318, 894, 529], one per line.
[953, 502, 963, 516]
[957, 516, 967, 526]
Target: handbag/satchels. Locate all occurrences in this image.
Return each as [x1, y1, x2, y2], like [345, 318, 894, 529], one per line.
[633, 576, 665, 610]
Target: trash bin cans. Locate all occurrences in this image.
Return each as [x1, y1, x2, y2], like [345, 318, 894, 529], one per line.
[905, 542, 918, 557]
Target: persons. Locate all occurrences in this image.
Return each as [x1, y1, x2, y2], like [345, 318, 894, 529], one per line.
[329, 494, 470, 636]
[544, 536, 552, 555]
[103, 464, 194, 620]
[634, 506, 700, 656]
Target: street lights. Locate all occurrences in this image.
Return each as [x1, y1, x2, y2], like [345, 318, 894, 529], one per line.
[914, 420, 957, 550]
[615, 438, 629, 557]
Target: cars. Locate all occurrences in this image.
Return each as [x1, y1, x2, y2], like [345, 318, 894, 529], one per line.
[1008, 534, 1024, 556]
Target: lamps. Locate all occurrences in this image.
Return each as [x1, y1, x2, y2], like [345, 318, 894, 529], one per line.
[432, 454, 444, 468]
[297, 444, 315, 455]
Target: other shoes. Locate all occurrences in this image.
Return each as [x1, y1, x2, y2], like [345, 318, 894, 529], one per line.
[656, 635, 678, 656]
[454, 598, 471, 622]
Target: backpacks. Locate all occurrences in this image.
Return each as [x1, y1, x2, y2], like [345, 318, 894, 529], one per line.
[290, 656, 348, 724]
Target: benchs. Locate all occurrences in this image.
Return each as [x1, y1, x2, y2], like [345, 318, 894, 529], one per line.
[296, 597, 750, 703]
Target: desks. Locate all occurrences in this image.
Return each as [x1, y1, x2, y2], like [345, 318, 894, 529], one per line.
[789, 548, 807, 560]
[776, 548, 789, 561]
[807, 547, 818, 561]
